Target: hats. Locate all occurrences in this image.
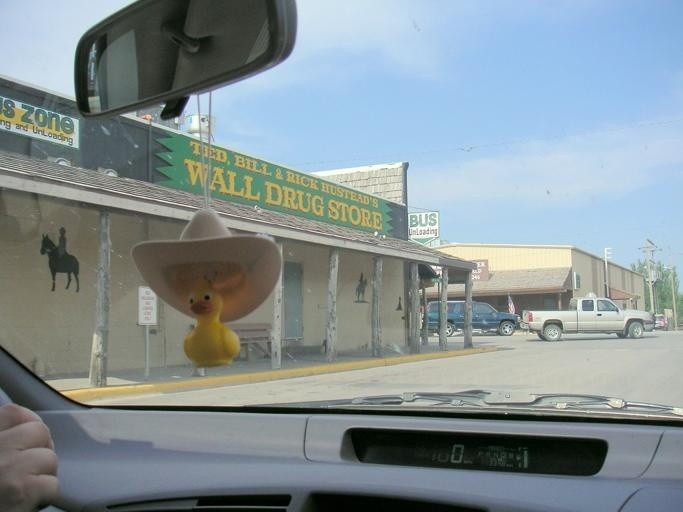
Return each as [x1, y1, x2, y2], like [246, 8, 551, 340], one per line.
[129, 206, 283, 324]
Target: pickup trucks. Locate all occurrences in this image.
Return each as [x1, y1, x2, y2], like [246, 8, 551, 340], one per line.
[521, 293, 659, 342]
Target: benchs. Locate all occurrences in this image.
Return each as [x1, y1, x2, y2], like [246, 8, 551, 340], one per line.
[191, 321, 272, 362]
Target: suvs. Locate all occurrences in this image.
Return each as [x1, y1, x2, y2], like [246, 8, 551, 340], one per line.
[421, 300, 523, 339]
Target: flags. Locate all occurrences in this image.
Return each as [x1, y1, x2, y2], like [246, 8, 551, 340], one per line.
[507, 296, 515, 314]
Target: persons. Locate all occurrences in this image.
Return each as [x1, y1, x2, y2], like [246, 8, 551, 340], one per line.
[1, 401, 59, 512]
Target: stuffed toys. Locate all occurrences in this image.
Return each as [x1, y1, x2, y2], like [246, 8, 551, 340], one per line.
[183, 275, 240, 369]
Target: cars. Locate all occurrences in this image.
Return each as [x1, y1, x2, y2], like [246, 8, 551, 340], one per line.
[652, 313, 670, 332]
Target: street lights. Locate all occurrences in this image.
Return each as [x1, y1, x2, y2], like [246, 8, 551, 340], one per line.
[604, 247, 614, 298]
